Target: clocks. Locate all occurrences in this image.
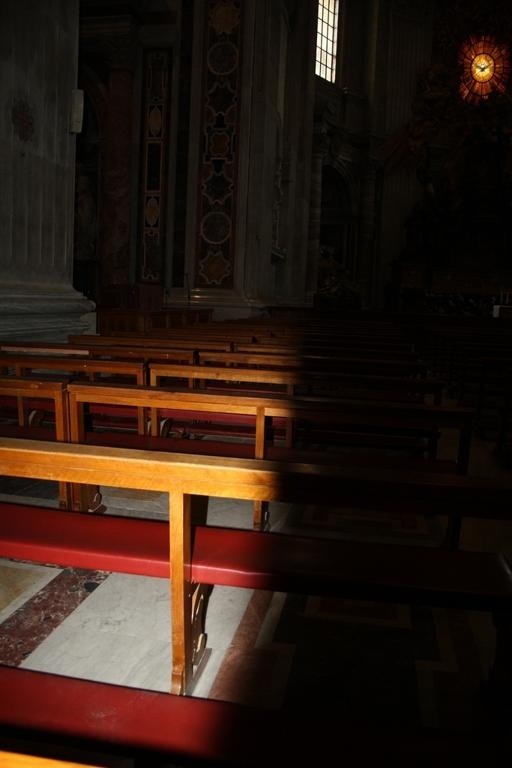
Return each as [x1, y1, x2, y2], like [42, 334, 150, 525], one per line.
[462, 40, 505, 96]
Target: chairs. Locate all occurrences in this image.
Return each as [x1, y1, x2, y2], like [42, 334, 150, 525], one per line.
[0, 306, 511, 767]
